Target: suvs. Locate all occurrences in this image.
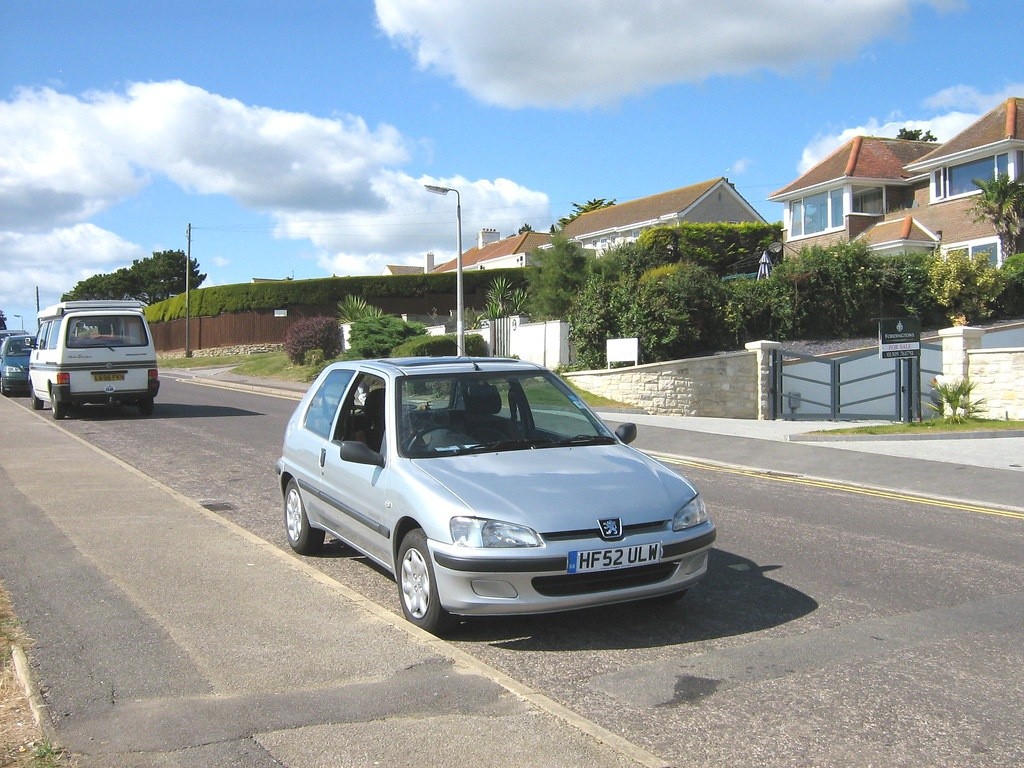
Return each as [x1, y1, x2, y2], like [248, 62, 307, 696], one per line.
[0, 329, 32, 355]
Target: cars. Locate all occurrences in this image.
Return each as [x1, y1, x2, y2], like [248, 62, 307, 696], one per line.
[275, 355, 717, 641]
[0, 334, 37, 397]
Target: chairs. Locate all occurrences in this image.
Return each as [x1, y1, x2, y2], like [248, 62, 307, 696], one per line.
[458, 384, 518, 442]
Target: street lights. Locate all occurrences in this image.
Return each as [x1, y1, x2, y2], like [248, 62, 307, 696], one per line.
[14, 315, 23, 330]
[425, 184, 466, 357]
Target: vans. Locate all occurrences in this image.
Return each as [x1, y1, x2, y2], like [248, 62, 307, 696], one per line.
[25, 300, 161, 420]
